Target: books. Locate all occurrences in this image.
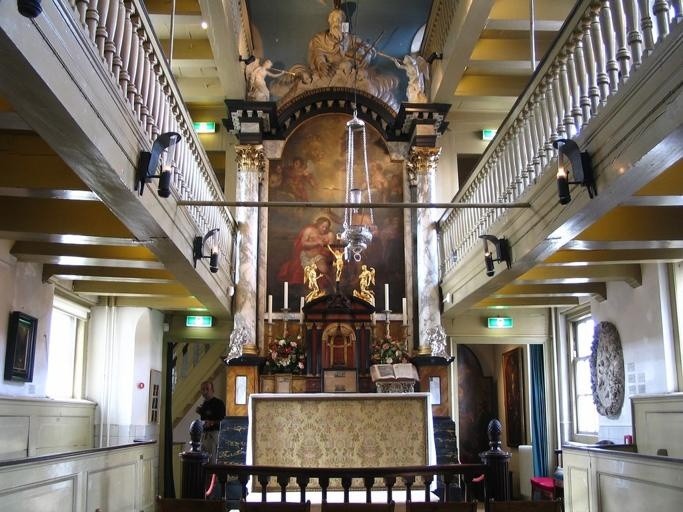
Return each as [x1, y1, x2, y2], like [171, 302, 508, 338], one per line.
[370, 363, 420, 383]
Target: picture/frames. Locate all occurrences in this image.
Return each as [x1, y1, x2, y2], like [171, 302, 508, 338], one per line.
[502, 346, 526, 448]
[4, 309, 39, 383]
[319, 367, 360, 394]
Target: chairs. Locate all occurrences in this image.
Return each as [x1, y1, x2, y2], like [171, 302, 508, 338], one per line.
[530, 450, 564, 499]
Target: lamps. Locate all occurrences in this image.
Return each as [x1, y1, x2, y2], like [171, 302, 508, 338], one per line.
[134, 129, 179, 200]
[480, 233, 512, 275]
[193, 225, 221, 274]
[553, 138, 594, 208]
[341, 22, 377, 264]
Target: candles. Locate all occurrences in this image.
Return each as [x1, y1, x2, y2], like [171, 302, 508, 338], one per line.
[372, 281, 407, 327]
[264, 277, 305, 326]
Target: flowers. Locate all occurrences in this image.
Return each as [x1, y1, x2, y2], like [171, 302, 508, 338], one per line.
[369, 335, 410, 365]
[269, 332, 305, 374]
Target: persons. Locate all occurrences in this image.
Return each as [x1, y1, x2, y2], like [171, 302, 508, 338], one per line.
[196, 381, 226, 465]
[392, 54, 424, 103]
[308, 7, 371, 74]
[269, 156, 403, 290]
[250, 59, 284, 100]
